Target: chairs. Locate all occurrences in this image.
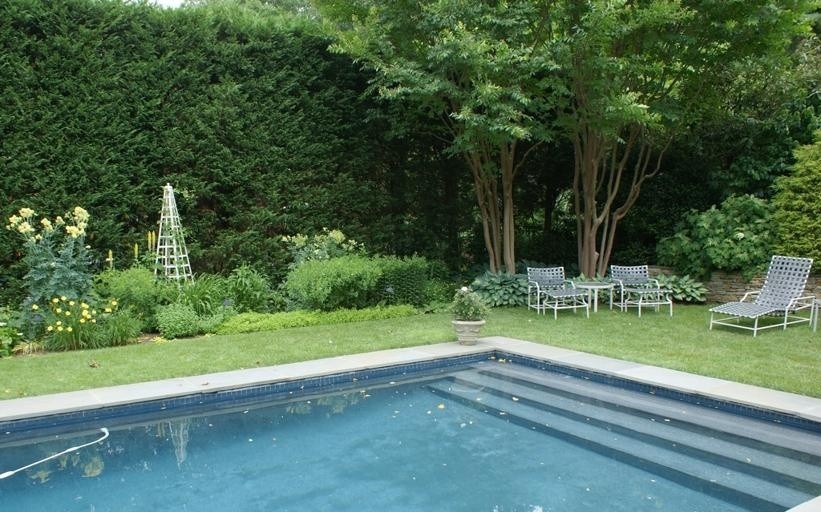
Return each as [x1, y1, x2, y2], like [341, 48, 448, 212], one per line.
[526, 263, 674, 321]
[708, 255, 816, 338]
